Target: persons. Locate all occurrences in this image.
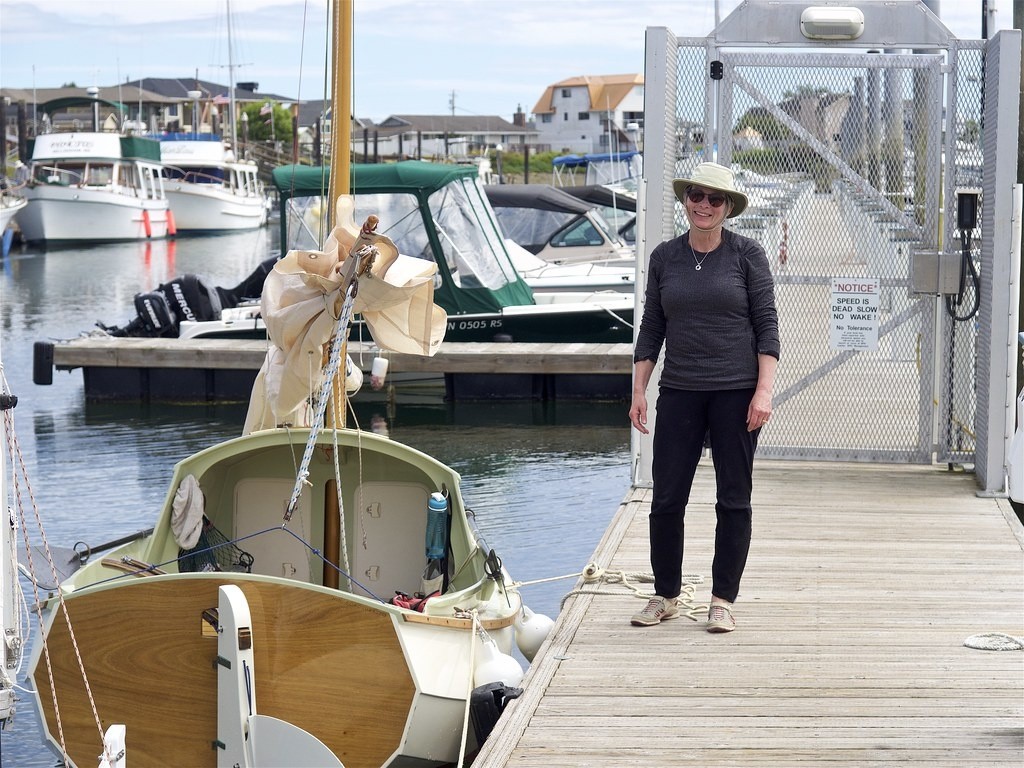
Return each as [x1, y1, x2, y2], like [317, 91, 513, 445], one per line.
[629, 162, 780, 632]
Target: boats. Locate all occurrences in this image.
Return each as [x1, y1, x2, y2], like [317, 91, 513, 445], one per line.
[7, 62, 175, 250]
[139, 0, 271, 237]
[480, 179, 639, 293]
[74, 159, 636, 411]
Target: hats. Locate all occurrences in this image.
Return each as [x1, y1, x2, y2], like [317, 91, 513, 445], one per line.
[672, 162, 748, 218]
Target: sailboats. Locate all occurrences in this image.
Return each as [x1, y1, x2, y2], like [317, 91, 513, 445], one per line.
[0, 0, 560, 766]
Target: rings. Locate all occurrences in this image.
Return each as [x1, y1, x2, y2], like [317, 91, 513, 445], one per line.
[763, 420, 768, 423]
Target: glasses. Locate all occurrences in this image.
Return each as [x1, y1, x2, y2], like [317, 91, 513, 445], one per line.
[688, 189, 726, 207]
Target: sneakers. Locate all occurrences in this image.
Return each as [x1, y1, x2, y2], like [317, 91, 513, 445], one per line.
[706, 602, 736, 631]
[631, 596, 680, 626]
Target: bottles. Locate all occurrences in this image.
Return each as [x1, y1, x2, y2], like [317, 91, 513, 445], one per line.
[425, 488, 449, 558]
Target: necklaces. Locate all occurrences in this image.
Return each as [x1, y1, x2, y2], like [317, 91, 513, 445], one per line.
[690, 230, 720, 270]
